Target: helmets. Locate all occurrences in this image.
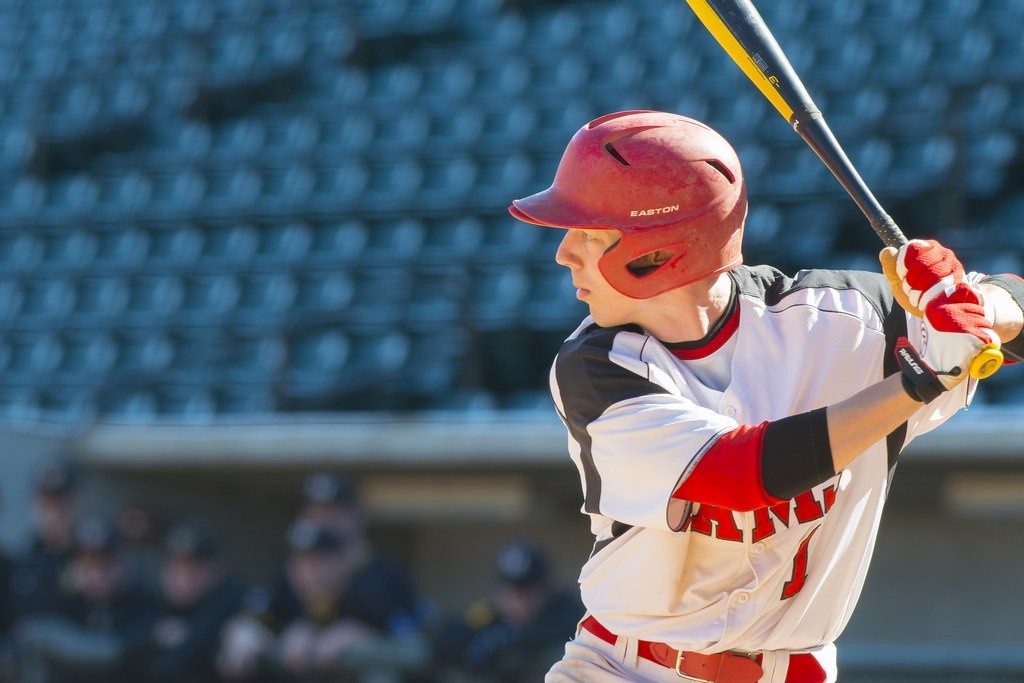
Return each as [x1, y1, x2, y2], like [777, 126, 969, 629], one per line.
[508, 109, 748, 299]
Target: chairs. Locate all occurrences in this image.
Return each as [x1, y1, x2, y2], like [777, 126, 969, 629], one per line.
[0, 0, 1024, 422]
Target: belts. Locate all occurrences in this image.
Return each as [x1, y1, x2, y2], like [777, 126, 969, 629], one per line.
[580, 615, 827, 683]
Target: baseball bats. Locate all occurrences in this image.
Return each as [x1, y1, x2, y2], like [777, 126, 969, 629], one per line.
[686, 0, 1004, 380]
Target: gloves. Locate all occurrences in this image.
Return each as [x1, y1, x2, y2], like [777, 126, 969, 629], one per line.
[894, 281, 1000, 405]
[878, 237, 969, 321]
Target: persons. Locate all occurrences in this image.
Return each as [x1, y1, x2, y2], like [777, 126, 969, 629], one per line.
[506, 110, 1024, 683]
